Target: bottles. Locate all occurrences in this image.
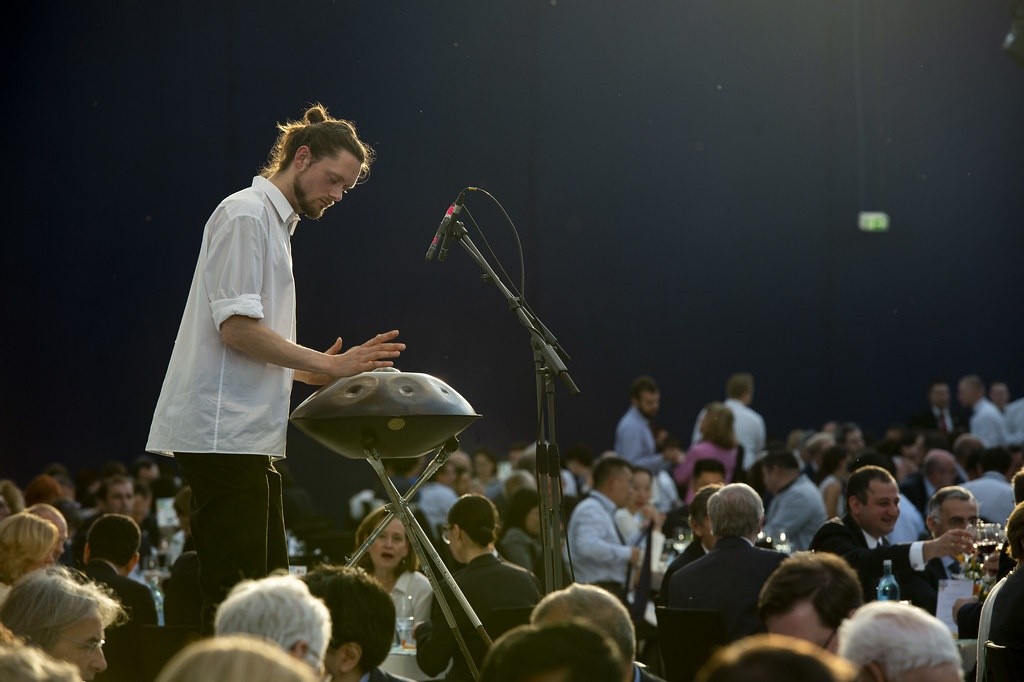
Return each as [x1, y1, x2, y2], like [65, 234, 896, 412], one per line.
[877, 559, 901, 600]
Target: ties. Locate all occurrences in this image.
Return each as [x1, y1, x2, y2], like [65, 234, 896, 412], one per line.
[940, 413, 947, 433]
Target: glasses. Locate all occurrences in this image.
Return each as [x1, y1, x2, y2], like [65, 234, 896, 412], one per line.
[442, 525, 463, 544]
[59, 633, 105, 657]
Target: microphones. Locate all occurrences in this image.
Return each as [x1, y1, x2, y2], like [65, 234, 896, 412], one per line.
[423, 192, 466, 263]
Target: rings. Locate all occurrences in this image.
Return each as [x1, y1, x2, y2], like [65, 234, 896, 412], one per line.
[951, 542, 954, 546]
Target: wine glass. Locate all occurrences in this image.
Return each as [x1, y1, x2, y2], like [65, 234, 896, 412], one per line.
[396, 593, 414, 647]
[946, 518, 1001, 595]
[142, 554, 162, 602]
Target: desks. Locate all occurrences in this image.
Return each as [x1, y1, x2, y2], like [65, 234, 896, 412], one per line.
[378, 645, 453, 681]
[935, 578, 973, 640]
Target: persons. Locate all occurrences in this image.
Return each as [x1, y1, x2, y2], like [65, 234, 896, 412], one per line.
[145, 106, 406, 640]
[1, 372, 1024, 682]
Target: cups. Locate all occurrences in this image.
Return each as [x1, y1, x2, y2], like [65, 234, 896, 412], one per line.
[775, 527, 789, 554]
[672, 526, 694, 553]
